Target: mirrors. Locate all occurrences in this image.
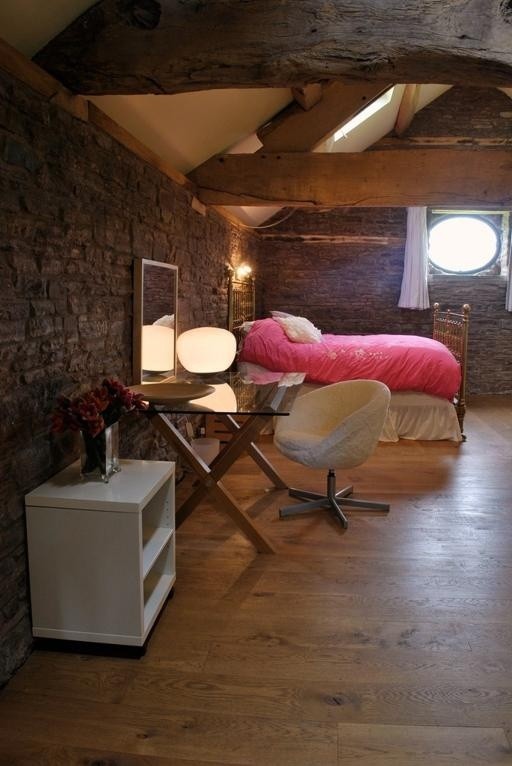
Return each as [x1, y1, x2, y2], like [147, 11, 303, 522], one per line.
[133, 258, 179, 384]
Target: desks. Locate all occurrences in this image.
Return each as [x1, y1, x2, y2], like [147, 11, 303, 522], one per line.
[124, 372, 306, 554]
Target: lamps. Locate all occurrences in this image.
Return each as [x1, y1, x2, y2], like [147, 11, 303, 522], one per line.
[142, 325, 175, 376]
[237, 262, 251, 279]
[177, 327, 238, 376]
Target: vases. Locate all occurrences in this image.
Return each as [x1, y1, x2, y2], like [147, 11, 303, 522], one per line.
[80, 421, 122, 483]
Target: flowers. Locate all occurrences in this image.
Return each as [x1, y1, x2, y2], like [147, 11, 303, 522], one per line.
[48, 376, 150, 439]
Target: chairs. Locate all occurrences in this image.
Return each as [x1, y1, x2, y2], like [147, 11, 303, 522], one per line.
[272, 379, 391, 528]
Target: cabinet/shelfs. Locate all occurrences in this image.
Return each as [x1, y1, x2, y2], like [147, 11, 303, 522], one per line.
[25, 460, 176, 647]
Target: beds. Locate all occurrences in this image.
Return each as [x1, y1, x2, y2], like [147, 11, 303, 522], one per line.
[228, 270, 471, 443]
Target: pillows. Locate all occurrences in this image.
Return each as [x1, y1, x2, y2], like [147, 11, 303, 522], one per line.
[272, 316, 322, 344]
[270, 310, 295, 319]
